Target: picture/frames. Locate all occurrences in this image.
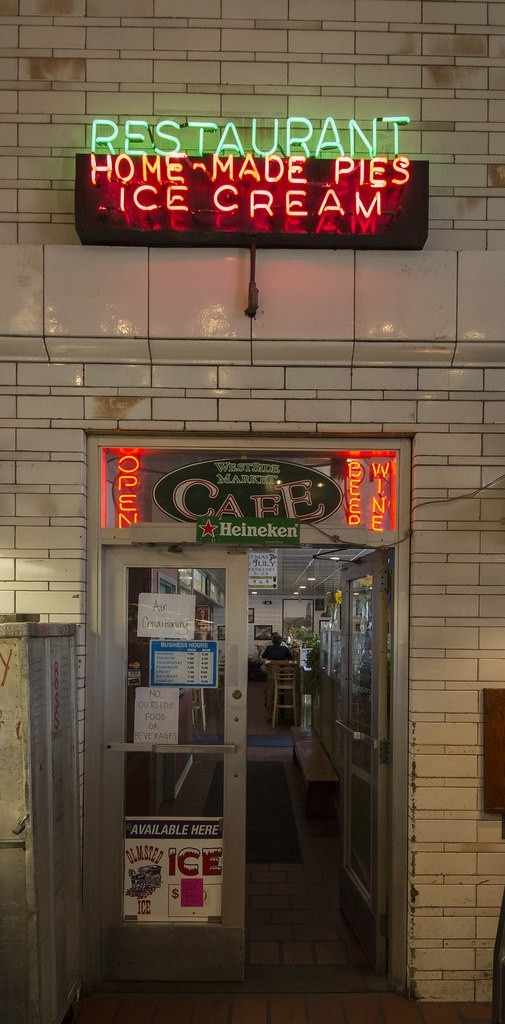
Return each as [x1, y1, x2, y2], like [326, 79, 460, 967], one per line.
[282, 598, 315, 640]
[248, 607, 254, 623]
[218, 625, 226, 640]
[254, 624, 272, 641]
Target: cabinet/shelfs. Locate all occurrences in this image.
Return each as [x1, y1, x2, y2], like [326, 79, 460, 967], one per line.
[178, 567, 225, 611]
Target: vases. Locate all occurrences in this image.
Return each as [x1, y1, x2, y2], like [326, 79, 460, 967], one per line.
[330, 608, 340, 630]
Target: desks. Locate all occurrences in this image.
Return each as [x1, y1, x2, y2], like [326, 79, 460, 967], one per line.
[264, 659, 299, 667]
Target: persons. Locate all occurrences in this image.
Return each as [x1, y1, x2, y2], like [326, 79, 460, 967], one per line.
[255, 632, 292, 661]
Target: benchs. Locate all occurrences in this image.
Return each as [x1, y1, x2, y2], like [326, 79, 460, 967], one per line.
[290, 727, 340, 817]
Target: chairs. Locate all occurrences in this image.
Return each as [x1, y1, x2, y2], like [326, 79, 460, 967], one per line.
[269, 665, 300, 730]
[192, 688, 207, 731]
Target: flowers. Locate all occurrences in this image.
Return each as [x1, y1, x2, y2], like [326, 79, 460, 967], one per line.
[325, 589, 342, 608]
